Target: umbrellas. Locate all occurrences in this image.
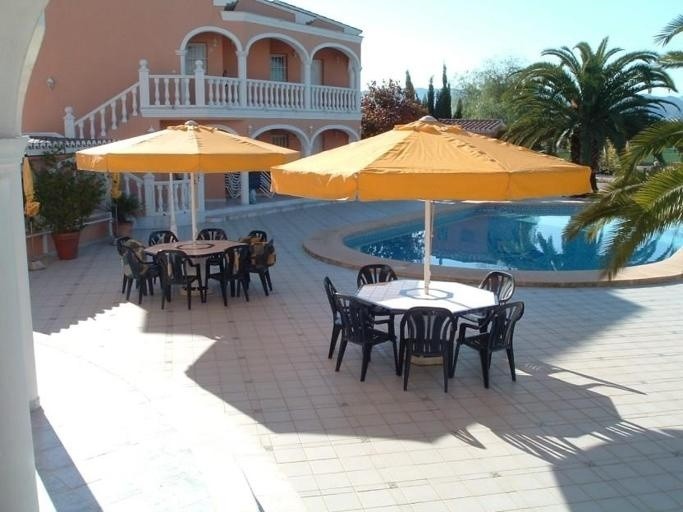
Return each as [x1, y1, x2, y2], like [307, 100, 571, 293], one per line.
[76, 120, 301, 246]
[22, 157, 41, 234]
[269, 114, 595, 294]
[111, 171, 122, 236]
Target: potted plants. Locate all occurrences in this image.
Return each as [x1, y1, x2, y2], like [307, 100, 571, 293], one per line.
[28, 152, 105, 260]
[108, 193, 140, 236]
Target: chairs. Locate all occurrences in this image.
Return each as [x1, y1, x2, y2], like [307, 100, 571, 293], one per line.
[322, 263, 525, 393]
[111, 228, 275, 311]
